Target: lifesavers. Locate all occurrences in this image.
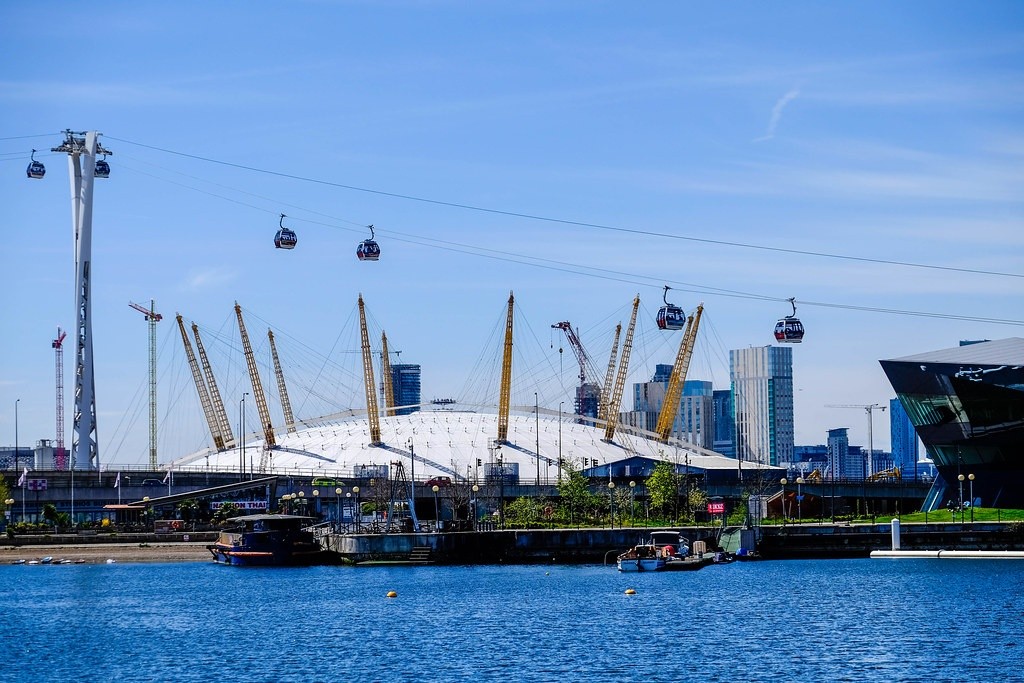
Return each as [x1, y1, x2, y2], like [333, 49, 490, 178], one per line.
[544, 506, 552, 515]
[171, 521, 179, 528]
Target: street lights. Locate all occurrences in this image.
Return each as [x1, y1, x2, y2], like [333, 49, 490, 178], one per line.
[433, 485, 440, 534]
[281, 489, 318, 516]
[559, 401, 565, 483]
[797, 477, 802, 524]
[335, 486, 359, 531]
[608, 481, 615, 528]
[15, 399, 21, 472]
[969, 474, 975, 520]
[958, 474, 964, 523]
[535, 392, 539, 485]
[629, 481, 636, 527]
[472, 485, 479, 530]
[781, 477, 787, 522]
[239, 392, 249, 483]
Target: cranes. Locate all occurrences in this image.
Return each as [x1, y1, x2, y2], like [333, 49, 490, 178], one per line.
[51, 327, 68, 470]
[127, 297, 163, 472]
[576, 328, 587, 398]
[825, 402, 887, 479]
[341, 349, 401, 416]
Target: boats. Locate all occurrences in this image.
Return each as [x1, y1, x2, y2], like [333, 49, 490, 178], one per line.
[617, 530, 690, 572]
[207, 513, 319, 567]
[713, 548, 761, 565]
